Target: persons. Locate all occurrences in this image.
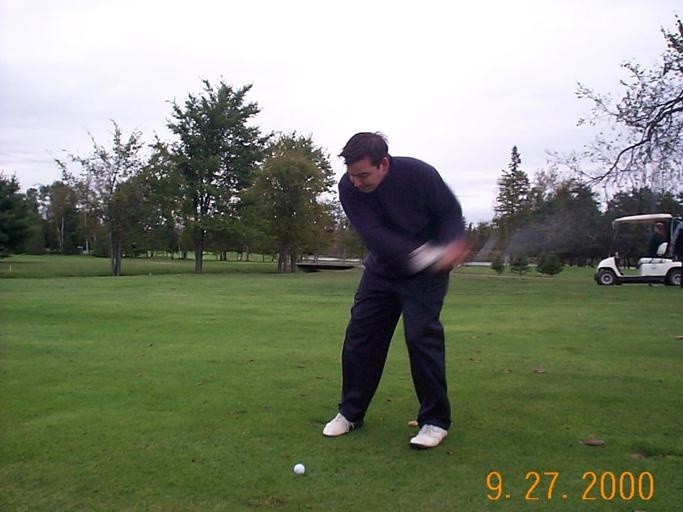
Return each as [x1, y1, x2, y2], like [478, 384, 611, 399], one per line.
[645, 222, 669, 257]
[323, 132, 469, 448]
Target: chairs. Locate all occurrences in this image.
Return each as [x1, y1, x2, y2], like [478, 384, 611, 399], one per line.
[637, 242, 673, 265]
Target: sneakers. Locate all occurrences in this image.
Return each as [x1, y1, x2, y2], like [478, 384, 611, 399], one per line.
[321, 410, 363, 438]
[407, 421, 449, 449]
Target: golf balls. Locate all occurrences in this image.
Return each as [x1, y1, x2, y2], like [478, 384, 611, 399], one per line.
[294, 464, 305, 474]
